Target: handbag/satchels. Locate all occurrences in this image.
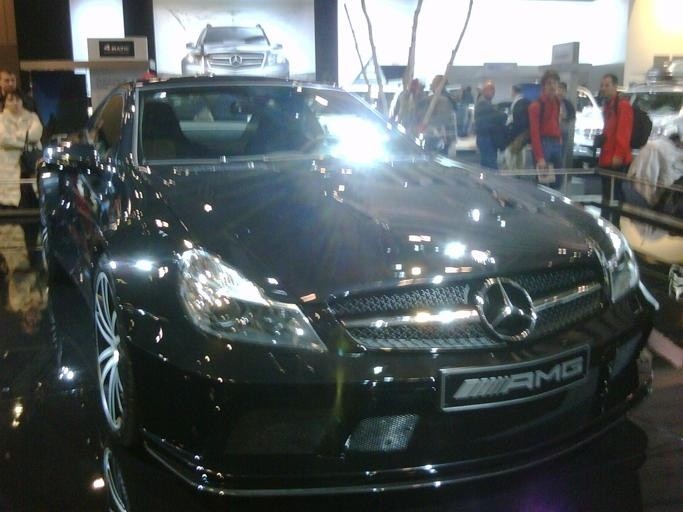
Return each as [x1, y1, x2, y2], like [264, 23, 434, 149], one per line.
[18, 131, 43, 209]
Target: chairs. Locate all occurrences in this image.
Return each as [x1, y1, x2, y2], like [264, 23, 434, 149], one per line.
[138, 96, 312, 161]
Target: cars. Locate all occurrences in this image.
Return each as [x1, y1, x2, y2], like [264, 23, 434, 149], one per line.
[575, 80, 683, 221]
[36, 76, 661, 500]
[181, 24, 288, 81]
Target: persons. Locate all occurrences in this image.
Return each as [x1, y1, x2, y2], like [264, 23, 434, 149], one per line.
[0, 86, 45, 211]
[1, 222, 53, 337]
[386, 70, 577, 191]
[594, 74, 636, 231]
[0, 65, 35, 115]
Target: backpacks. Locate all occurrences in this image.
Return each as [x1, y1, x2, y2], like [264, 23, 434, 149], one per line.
[614, 95, 653, 150]
